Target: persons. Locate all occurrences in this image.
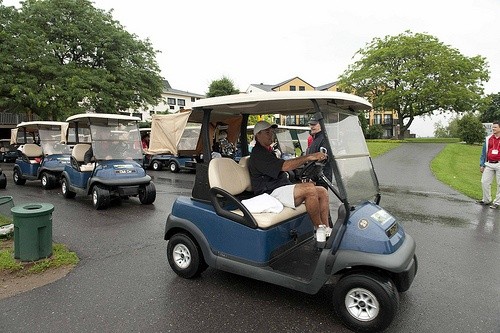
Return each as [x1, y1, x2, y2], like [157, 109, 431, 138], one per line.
[301, 114, 333, 233]
[476, 121, 500, 209]
[133, 134, 148, 157]
[249, 120, 333, 241]
[84, 126, 121, 166]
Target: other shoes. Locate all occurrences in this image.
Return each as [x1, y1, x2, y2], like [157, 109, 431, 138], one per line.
[491, 204, 498, 209]
[476, 200, 492, 205]
[313, 225, 332, 242]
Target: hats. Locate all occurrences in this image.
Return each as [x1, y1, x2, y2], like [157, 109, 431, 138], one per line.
[307, 114, 319, 124]
[253, 121, 278, 134]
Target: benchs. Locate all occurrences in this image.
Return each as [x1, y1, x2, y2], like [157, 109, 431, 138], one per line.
[70, 144, 99, 172]
[21, 144, 63, 165]
[208, 156, 307, 230]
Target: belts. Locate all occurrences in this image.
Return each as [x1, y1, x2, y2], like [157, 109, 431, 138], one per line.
[487, 160, 498, 163]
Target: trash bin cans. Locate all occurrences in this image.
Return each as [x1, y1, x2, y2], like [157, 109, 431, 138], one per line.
[10, 202, 56, 262]
[0, 195, 16, 242]
